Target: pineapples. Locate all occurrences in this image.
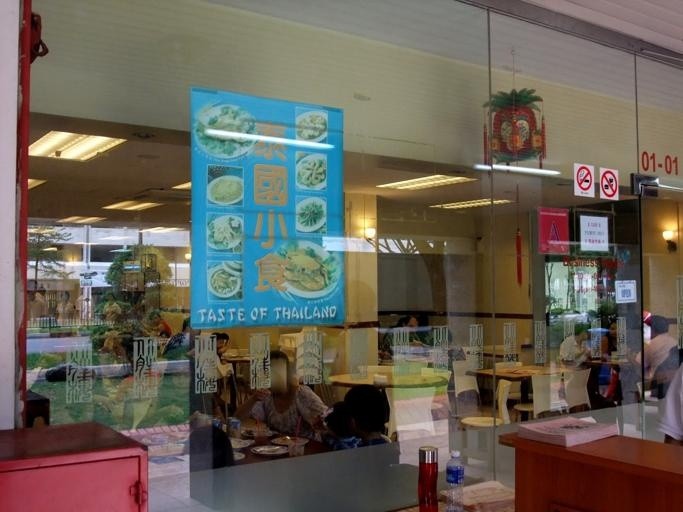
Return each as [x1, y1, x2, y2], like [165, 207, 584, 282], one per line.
[483, 88, 542, 152]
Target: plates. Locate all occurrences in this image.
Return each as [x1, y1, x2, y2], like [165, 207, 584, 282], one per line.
[295, 109, 328, 142]
[242, 429, 276, 437]
[273, 239, 338, 299]
[206, 213, 243, 252]
[251, 445, 288, 455]
[192, 105, 258, 158]
[206, 174, 244, 203]
[271, 435, 310, 447]
[295, 197, 325, 233]
[295, 153, 327, 189]
[206, 267, 241, 299]
[229, 451, 245, 460]
[228, 438, 249, 448]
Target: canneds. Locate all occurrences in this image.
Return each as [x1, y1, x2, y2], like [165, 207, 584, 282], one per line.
[213, 419, 222, 429]
[227, 418, 240, 437]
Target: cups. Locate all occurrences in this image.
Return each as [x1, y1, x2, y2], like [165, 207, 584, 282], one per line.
[287, 439, 304, 456]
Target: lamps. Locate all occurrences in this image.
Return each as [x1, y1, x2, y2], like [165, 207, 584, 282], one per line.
[663, 231, 676, 252]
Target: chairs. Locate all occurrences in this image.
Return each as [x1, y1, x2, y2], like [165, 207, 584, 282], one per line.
[650, 348, 683, 399]
[366, 364, 593, 466]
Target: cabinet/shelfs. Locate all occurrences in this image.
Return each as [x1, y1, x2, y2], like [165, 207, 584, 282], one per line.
[0, 423, 148, 512]
[23, 390, 49, 428]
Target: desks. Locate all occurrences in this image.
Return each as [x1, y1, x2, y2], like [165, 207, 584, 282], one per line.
[499, 431, 683, 511]
[326, 373, 447, 388]
[220, 352, 281, 416]
[588, 358, 631, 406]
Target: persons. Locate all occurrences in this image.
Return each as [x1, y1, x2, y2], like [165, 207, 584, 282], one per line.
[25, 279, 391, 472]
[447, 332, 466, 391]
[559, 315, 682, 445]
[380, 316, 421, 360]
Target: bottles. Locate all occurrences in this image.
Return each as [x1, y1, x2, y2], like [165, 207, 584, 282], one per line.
[415, 445, 440, 511]
[447, 449, 467, 511]
